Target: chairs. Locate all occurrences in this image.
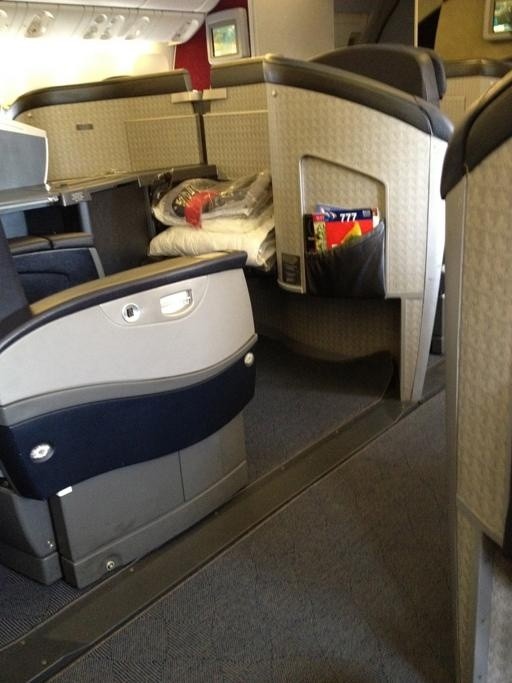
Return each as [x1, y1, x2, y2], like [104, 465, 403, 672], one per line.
[0, 207, 257, 592]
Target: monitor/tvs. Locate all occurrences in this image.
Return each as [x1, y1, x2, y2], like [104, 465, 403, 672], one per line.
[484, 1, 512, 41]
[206, 8, 251, 65]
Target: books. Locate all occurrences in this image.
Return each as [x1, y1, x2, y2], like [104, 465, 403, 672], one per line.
[303, 204, 381, 258]
